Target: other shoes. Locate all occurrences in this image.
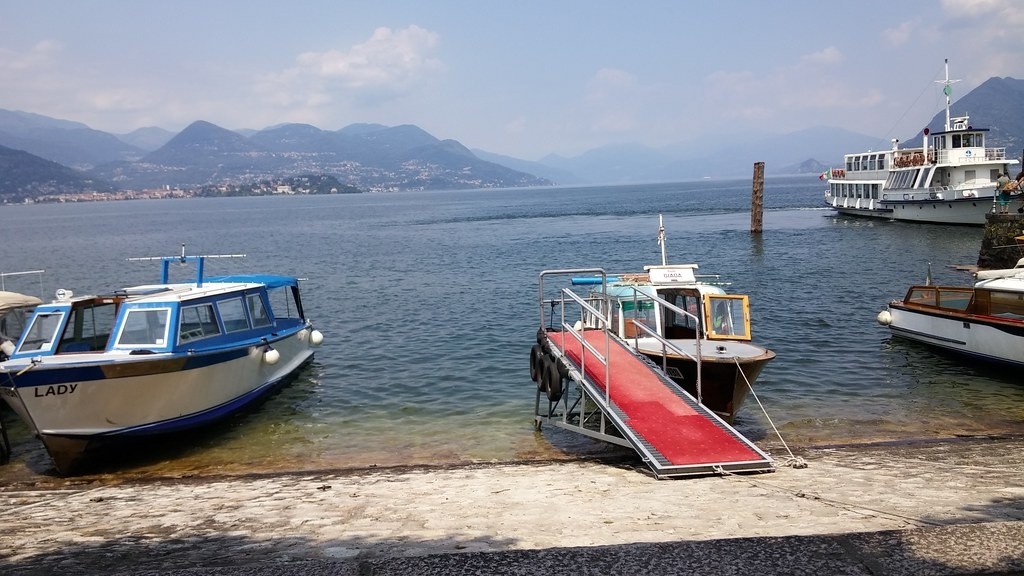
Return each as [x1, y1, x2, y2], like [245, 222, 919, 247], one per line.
[999, 212, 1003, 214]
[1005, 212, 1008, 214]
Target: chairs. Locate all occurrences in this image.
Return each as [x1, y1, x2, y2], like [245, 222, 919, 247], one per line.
[621, 317, 646, 337]
[64, 342, 91, 351]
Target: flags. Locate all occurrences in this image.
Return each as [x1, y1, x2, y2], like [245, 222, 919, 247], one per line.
[819, 169, 831, 181]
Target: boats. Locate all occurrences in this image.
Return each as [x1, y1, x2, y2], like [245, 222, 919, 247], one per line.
[819, 58, 1024, 224]
[591, 214, 776, 426]
[0, 243, 323, 479]
[877, 258, 1024, 366]
[0, 269, 45, 362]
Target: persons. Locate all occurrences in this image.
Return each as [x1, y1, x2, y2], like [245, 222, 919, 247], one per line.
[712, 302, 735, 334]
[997, 173, 1011, 214]
